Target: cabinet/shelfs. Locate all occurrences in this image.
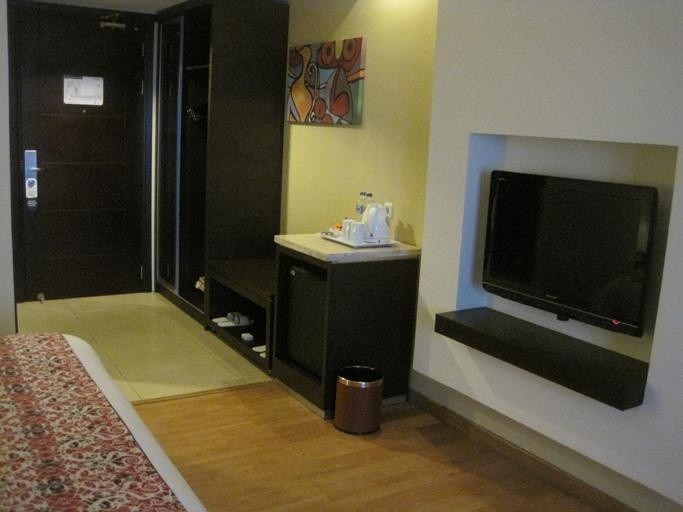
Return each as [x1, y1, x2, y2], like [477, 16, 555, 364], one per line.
[272, 233, 421, 420]
[156, 0, 289, 327]
[204, 259, 278, 375]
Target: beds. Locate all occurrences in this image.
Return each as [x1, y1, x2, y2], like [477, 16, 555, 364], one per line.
[0, 332, 208, 512]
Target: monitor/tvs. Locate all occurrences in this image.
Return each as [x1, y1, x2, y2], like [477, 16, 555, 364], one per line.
[482, 169, 658, 337]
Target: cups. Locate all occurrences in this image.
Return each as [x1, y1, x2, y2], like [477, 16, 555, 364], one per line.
[342, 219, 353, 240]
[351, 222, 363, 243]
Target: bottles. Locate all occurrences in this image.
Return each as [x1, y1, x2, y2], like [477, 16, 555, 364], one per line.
[355, 192, 366, 220]
[365, 194, 372, 205]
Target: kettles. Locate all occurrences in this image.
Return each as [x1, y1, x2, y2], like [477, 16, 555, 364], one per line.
[361, 202, 392, 242]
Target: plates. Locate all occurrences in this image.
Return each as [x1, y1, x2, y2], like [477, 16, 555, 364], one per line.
[323, 235, 399, 248]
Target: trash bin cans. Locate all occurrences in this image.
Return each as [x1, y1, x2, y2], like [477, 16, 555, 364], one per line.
[333, 365, 385, 435]
